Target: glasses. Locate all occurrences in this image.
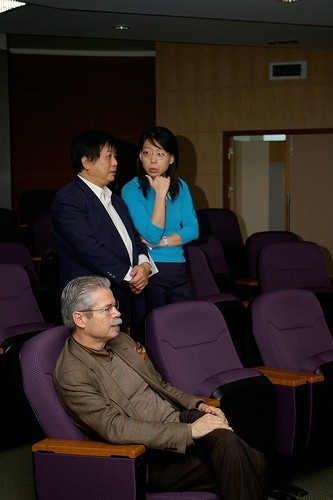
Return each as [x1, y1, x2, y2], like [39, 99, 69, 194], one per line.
[140, 147, 173, 160]
[77, 301, 119, 315]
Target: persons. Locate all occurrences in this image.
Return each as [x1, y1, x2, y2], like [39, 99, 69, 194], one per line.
[52, 128, 152, 338]
[121, 126, 200, 317]
[54, 275, 267, 500]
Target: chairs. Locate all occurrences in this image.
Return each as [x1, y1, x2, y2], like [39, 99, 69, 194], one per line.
[17, 324, 222, 497]
[250, 290, 331, 383]
[149, 299, 308, 466]
[1, 208, 332, 350]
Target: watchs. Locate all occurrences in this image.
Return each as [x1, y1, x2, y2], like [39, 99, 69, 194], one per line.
[161, 236, 168, 246]
[145, 263, 152, 275]
